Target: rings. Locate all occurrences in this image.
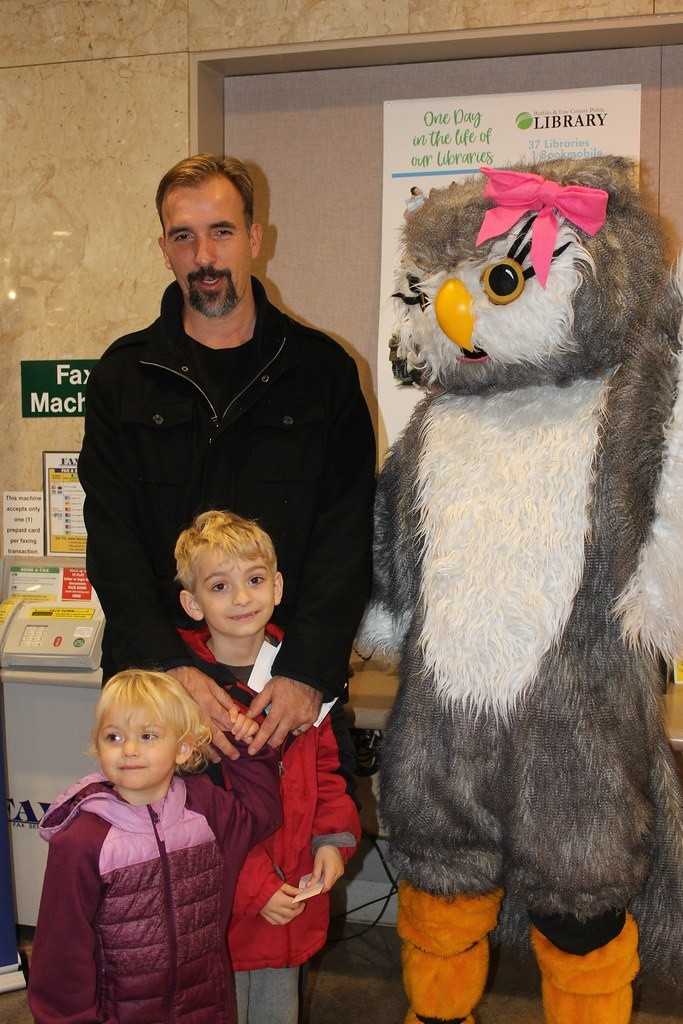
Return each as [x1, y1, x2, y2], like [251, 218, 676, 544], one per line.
[297, 728, 305, 735]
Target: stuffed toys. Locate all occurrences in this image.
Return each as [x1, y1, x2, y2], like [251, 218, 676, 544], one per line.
[354, 154, 683, 1024]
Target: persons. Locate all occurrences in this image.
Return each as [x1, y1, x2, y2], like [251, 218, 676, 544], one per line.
[27, 671, 284, 1024]
[177, 511, 361, 1024]
[77, 153, 378, 816]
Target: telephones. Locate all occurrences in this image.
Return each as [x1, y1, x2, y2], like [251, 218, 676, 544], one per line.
[0, 596, 106, 671]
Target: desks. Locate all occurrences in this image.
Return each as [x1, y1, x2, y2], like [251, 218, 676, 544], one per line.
[346, 640, 683, 752]
